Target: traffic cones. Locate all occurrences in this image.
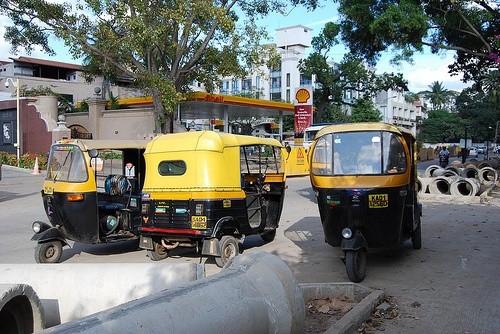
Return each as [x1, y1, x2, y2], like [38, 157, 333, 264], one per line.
[30, 157, 40, 176]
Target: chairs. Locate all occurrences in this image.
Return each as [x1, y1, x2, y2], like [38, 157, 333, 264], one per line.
[98, 174, 132, 209]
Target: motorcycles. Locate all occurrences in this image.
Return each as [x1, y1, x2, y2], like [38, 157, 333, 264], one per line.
[304, 120, 423, 283]
[30, 138, 150, 264]
[137, 130, 291, 270]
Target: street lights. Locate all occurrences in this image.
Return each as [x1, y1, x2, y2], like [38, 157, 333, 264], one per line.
[383, 96, 397, 122]
[5, 77, 21, 167]
[486, 126, 492, 160]
[462, 102, 468, 164]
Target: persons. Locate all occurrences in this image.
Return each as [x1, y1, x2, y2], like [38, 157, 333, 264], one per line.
[498, 144, 500, 155]
[439, 146, 450, 165]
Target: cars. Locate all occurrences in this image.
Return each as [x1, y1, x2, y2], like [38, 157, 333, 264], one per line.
[457, 146, 488, 158]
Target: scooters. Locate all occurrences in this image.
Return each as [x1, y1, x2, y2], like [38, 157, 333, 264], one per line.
[439, 154, 449, 168]
